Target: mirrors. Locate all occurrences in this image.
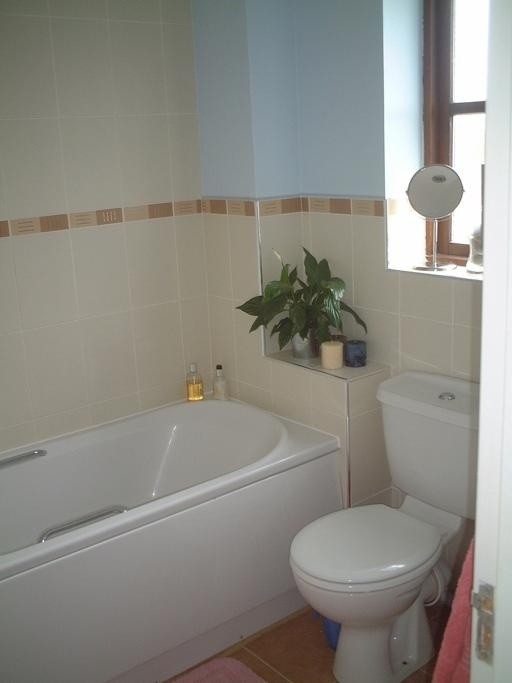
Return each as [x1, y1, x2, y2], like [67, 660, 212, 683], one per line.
[405, 163, 466, 273]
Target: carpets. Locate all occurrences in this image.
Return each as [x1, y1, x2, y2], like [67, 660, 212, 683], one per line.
[166, 653, 268, 683]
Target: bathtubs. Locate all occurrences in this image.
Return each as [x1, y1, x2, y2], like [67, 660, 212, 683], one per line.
[0, 394, 339, 581]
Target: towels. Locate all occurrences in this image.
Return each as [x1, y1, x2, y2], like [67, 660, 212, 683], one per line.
[430, 534, 473, 682]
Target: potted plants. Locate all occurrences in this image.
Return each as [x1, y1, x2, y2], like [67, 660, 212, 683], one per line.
[235, 245, 368, 360]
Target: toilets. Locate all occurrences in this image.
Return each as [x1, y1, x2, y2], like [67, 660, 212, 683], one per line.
[291, 371, 478, 681]
